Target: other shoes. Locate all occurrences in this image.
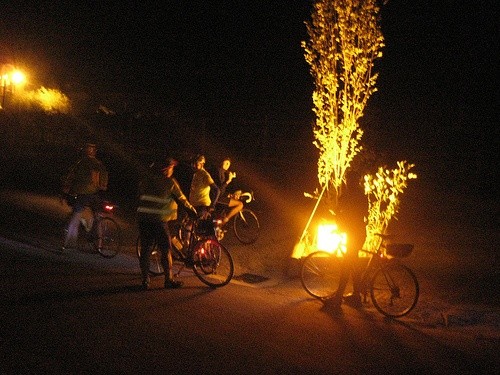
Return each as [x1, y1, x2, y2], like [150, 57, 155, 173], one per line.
[320, 295, 341, 305]
[342, 292, 362, 304]
[142, 279, 153, 289]
[164, 279, 184, 288]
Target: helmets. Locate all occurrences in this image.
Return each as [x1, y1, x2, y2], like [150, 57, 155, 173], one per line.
[191, 154, 205, 163]
[82, 143, 96, 155]
[161, 159, 176, 168]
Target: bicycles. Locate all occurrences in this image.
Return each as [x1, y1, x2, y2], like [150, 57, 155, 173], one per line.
[299, 233, 420, 318]
[136, 206, 234, 288]
[65, 205, 123, 258]
[178, 191, 260, 250]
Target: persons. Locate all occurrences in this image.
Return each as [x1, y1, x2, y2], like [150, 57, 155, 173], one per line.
[186, 152, 244, 249]
[321, 169, 369, 307]
[135, 157, 198, 289]
[59, 143, 109, 251]
